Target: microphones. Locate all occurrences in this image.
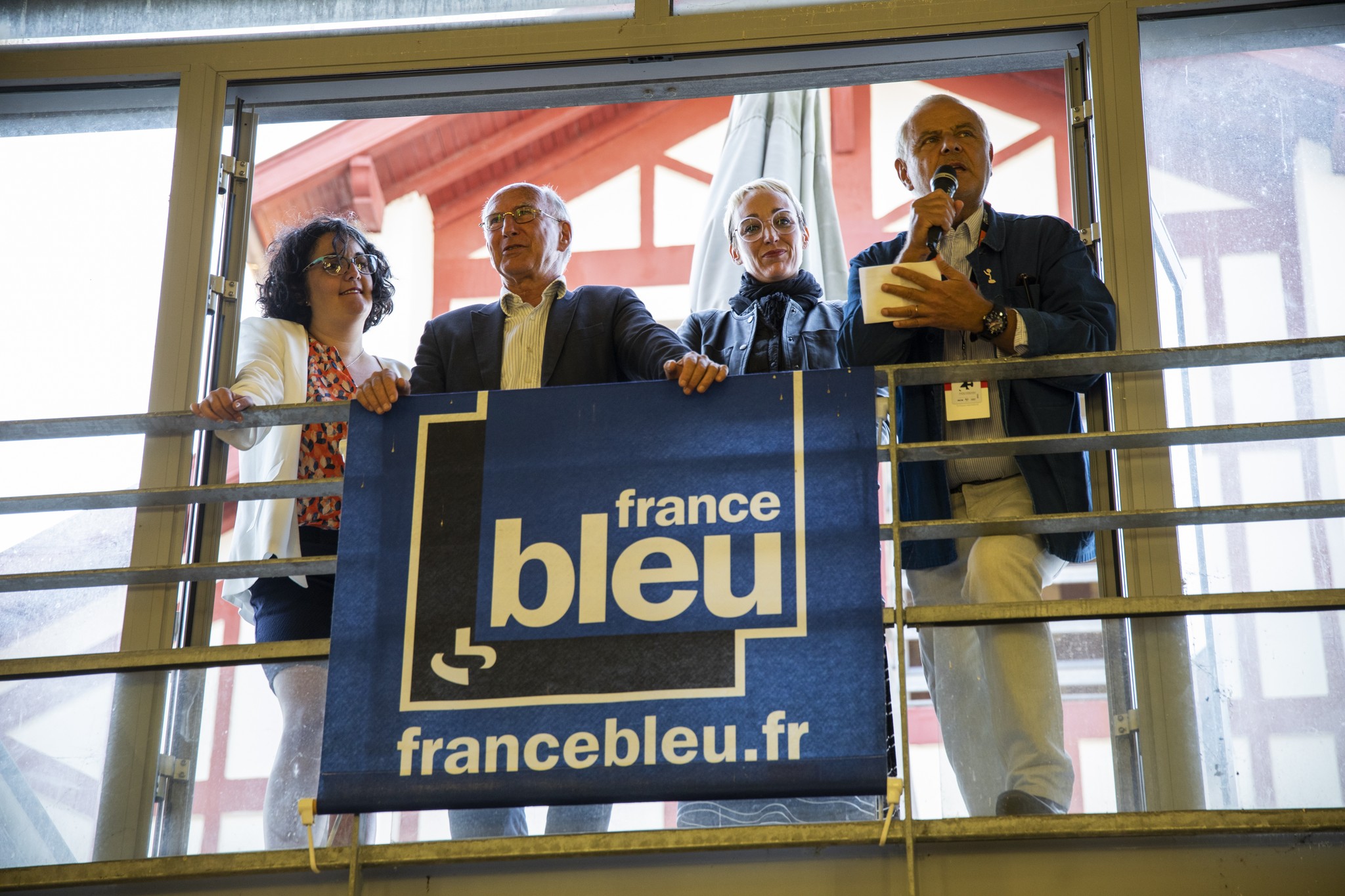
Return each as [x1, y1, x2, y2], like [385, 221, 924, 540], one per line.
[924, 164, 961, 257]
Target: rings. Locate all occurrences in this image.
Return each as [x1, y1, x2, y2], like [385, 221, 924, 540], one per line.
[915, 304, 919, 318]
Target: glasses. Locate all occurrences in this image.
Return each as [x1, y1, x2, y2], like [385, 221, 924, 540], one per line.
[479, 206, 562, 231]
[732, 209, 802, 242]
[297, 255, 378, 276]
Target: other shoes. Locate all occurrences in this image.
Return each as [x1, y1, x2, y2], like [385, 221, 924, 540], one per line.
[995, 789, 1066, 815]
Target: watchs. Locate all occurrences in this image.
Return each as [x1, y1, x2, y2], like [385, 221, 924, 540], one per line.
[969, 303, 1008, 342]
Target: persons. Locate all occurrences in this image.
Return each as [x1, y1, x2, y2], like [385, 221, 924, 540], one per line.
[675, 179, 898, 828]
[835, 93, 1117, 817]
[183, 216, 416, 853]
[345, 182, 729, 840]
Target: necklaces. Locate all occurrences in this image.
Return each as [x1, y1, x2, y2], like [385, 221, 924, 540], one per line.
[345, 348, 364, 368]
